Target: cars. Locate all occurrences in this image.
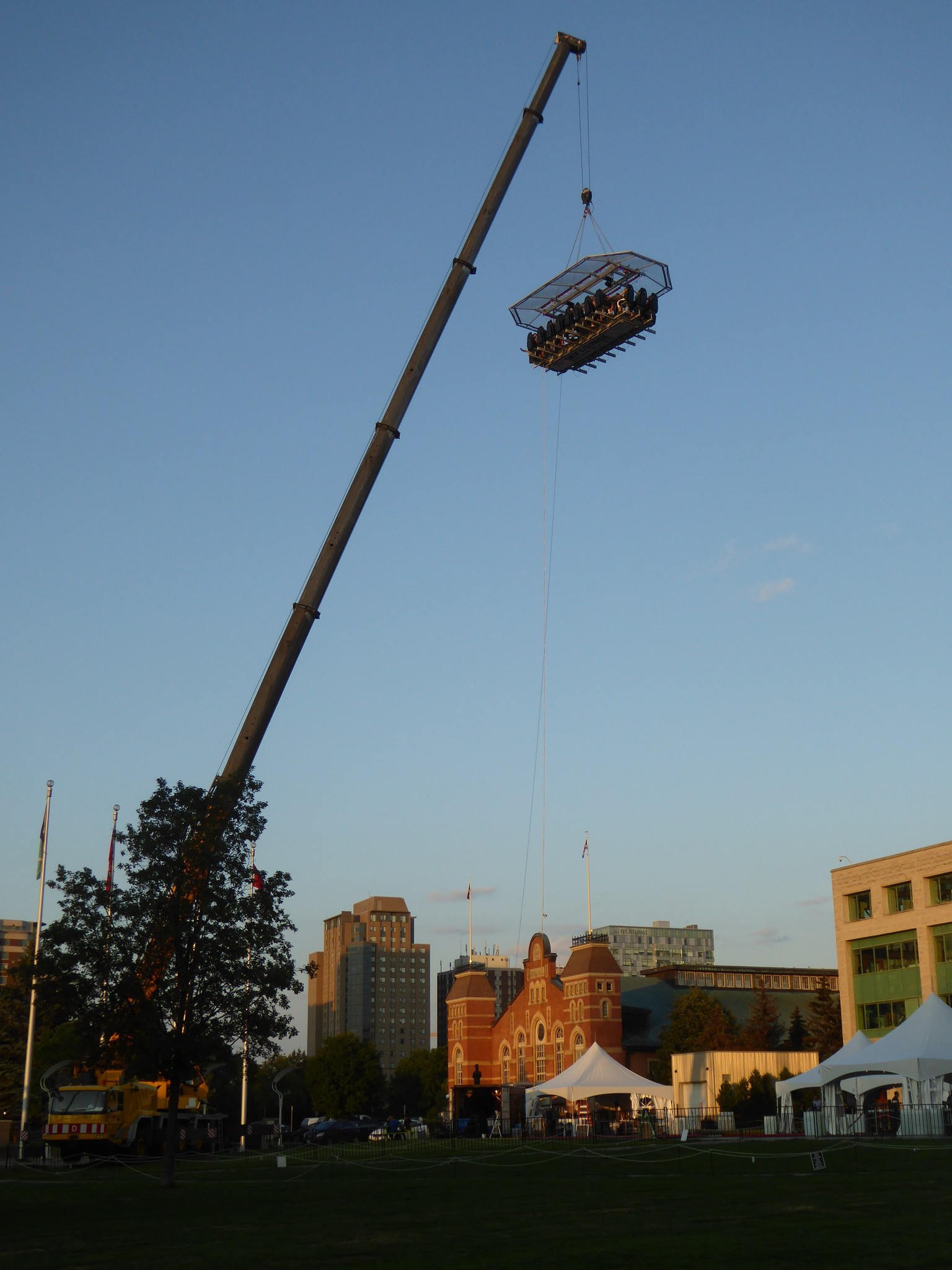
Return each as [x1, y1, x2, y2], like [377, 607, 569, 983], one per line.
[289, 1114, 430, 1145]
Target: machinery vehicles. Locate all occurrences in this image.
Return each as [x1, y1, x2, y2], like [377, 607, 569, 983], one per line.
[38, 30, 680, 1153]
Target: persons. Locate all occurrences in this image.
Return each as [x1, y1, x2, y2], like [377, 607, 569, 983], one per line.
[385, 1115, 423, 1141]
[533, 286, 652, 358]
[794, 1091, 901, 1135]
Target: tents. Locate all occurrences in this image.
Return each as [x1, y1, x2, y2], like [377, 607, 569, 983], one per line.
[819, 993, 952, 1135]
[775, 1029, 904, 1134]
[524, 1041, 677, 1136]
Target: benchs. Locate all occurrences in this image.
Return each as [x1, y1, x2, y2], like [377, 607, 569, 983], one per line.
[382, 1128, 414, 1141]
